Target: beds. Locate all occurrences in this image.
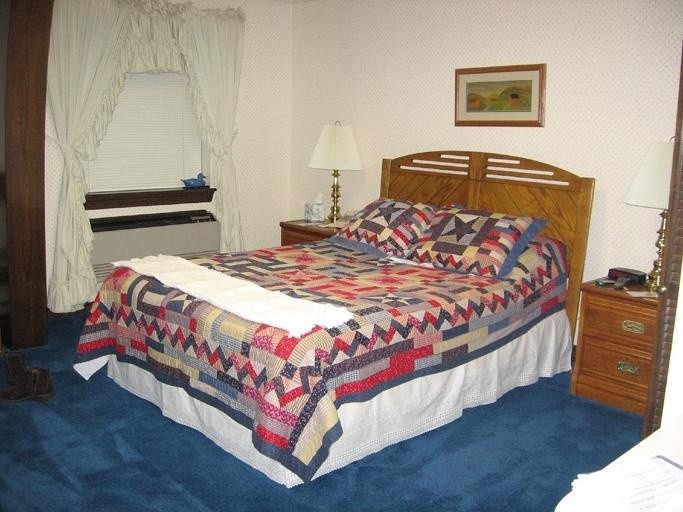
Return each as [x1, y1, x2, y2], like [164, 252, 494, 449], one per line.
[71, 149, 595, 489]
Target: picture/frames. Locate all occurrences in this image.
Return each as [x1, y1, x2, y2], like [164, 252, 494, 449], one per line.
[453, 62, 546, 128]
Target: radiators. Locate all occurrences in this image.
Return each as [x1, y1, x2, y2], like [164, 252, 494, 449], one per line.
[79, 209, 222, 305]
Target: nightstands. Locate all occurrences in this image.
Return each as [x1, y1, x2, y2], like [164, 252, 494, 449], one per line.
[279, 218, 340, 246]
[568, 275, 660, 418]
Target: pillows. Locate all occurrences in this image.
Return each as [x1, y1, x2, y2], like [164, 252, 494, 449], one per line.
[324, 196, 438, 261]
[385, 203, 547, 280]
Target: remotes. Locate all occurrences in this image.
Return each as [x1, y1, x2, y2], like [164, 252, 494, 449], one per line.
[615, 277, 627, 288]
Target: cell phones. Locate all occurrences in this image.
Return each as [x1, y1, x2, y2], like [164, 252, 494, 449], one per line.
[595, 279, 615, 286]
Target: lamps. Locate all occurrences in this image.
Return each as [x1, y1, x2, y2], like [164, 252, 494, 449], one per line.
[306, 121, 361, 223]
[621, 135, 675, 290]
